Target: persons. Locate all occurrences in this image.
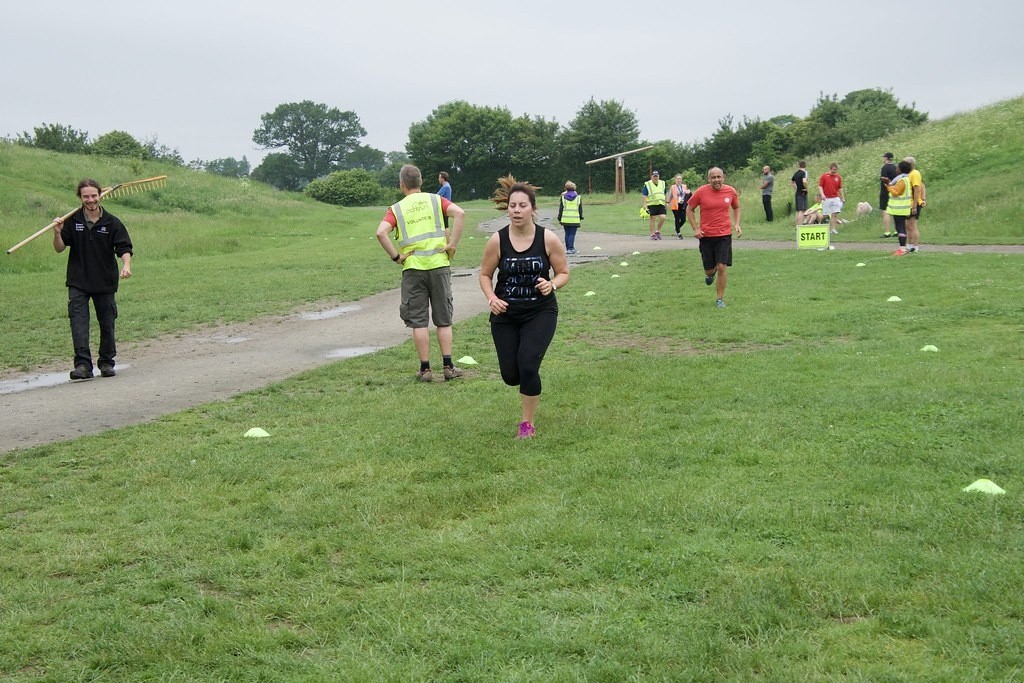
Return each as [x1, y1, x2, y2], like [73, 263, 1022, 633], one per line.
[479, 173, 569, 439]
[53, 179, 133, 380]
[376, 164, 465, 381]
[642, 170, 668, 240]
[819, 163, 845, 234]
[759, 165, 774, 222]
[666, 175, 690, 240]
[436, 171, 452, 244]
[557, 181, 584, 254]
[886, 162, 912, 256]
[791, 161, 808, 225]
[905, 157, 926, 254]
[686, 168, 743, 308]
[880, 152, 898, 238]
[804, 194, 831, 224]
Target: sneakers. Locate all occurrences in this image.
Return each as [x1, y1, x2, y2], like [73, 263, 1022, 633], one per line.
[70, 364, 94, 380]
[443, 366, 464, 379]
[101, 366, 115, 377]
[517, 421, 535, 439]
[417, 369, 432, 382]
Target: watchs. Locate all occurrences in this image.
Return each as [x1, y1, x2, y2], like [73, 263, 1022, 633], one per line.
[551, 282, 556, 291]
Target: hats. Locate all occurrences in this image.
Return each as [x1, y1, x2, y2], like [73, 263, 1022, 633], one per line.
[881, 153, 893, 158]
[652, 170, 659, 176]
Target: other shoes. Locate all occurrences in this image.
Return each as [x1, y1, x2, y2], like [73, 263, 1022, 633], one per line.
[909, 248, 919, 253]
[706, 268, 718, 285]
[893, 249, 909, 256]
[716, 299, 726, 307]
[830, 229, 839, 234]
[892, 232, 899, 237]
[655, 231, 663, 239]
[880, 233, 892, 238]
[675, 227, 683, 240]
[566, 249, 580, 254]
[651, 234, 657, 239]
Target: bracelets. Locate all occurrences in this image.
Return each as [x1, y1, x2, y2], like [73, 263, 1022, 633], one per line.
[922, 199, 925, 201]
[393, 254, 400, 261]
[488, 296, 497, 305]
